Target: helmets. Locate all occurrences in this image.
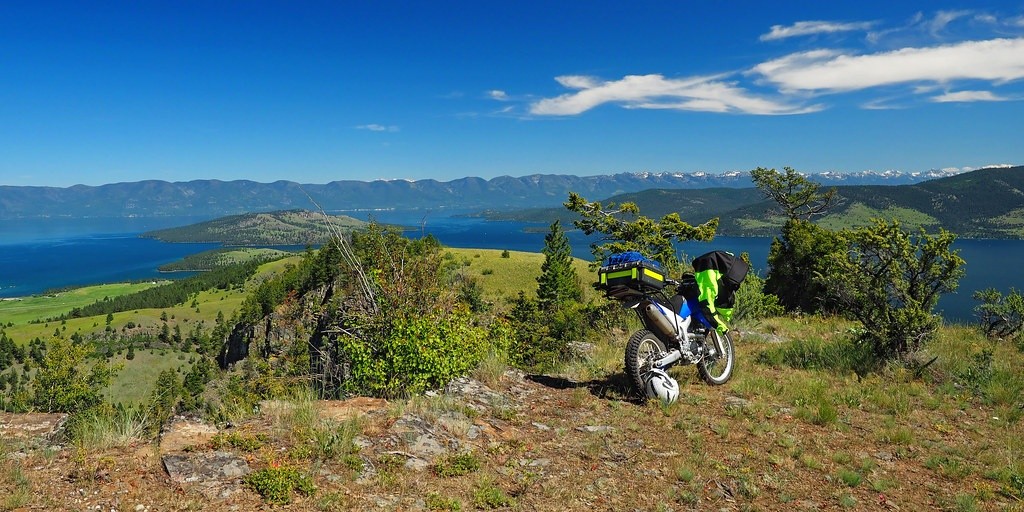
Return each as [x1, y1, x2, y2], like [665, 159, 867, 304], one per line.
[643, 369, 679, 407]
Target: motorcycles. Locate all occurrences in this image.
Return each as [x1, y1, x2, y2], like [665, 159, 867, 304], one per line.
[592, 252, 735, 403]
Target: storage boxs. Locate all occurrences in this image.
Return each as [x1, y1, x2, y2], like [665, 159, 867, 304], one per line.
[598, 263, 664, 290]
